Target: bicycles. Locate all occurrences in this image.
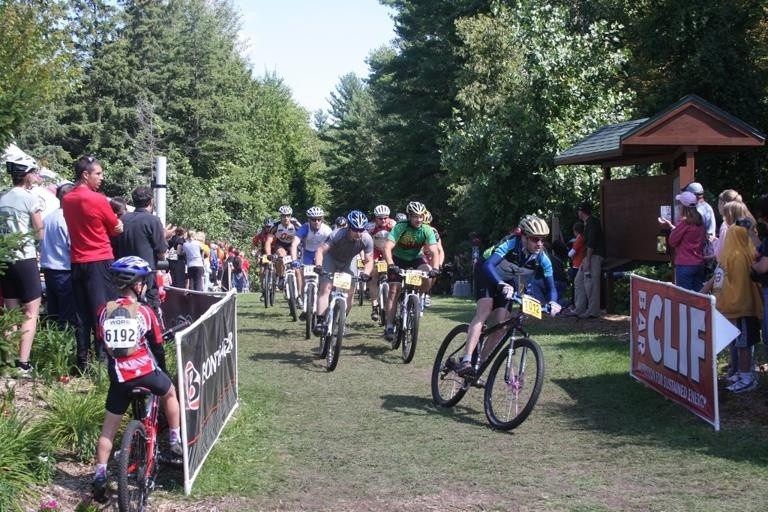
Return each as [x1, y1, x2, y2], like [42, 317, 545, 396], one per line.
[261, 253, 441, 374]
[109, 315, 202, 512]
[430, 286, 565, 431]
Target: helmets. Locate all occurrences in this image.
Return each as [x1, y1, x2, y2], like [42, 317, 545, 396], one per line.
[7, 155, 37, 175]
[306, 207, 324, 220]
[336, 201, 432, 229]
[520, 215, 550, 237]
[110, 256, 152, 289]
[280, 205, 293, 215]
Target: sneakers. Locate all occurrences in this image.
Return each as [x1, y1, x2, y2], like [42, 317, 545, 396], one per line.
[726, 371, 757, 393]
[171, 444, 183, 465]
[16, 360, 34, 373]
[458, 361, 472, 376]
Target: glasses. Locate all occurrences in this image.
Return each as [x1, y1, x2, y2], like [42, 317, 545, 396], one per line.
[352, 228, 364, 232]
[530, 237, 545, 242]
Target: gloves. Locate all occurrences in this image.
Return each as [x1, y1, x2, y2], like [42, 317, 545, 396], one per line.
[314, 265, 323, 274]
[429, 268, 439, 278]
[292, 261, 300, 270]
[549, 301, 562, 317]
[362, 273, 371, 281]
[497, 282, 514, 301]
[388, 264, 398, 273]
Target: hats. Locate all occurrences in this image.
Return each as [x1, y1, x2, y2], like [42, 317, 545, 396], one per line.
[675, 183, 704, 207]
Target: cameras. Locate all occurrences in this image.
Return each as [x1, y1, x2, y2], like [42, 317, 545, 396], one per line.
[735, 218, 752, 228]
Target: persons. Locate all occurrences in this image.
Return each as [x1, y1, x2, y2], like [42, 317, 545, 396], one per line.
[40, 183, 77, 331]
[658, 182, 768, 393]
[252, 201, 445, 335]
[457, 214, 562, 379]
[165, 226, 249, 293]
[91, 256, 183, 503]
[63, 155, 124, 377]
[564, 202, 606, 319]
[1, 154, 46, 378]
[110, 186, 176, 322]
